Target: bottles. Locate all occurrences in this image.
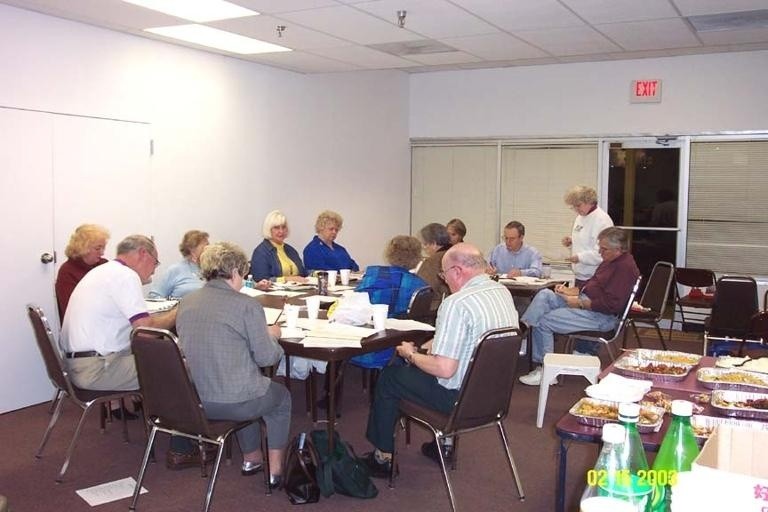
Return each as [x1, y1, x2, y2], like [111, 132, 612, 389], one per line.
[245, 275, 255, 295]
[540, 263, 552, 281]
[578, 397, 698, 512]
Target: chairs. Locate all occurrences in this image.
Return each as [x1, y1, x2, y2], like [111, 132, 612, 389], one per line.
[560, 262, 767, 384]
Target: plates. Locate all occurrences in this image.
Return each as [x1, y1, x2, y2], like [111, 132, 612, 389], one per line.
[569, 348, 768, 442]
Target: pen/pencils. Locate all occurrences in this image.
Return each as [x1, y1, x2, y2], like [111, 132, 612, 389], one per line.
[274, 312, 282, 325]
[559, 282, 566, 289]
[490, 261, 495, 269]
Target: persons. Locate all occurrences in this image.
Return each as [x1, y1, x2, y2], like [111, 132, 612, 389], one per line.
[55, 225, 141, 418]
[59, 234, 179, 390]
[363, 242, 519, 478]
[302, 211, 360, 271]
[418, 219, 466, 311]
[177, 242, 292, 487]
[558, 186, 620, 358]
[251, 212, 307, 282]
[490, 221, 545, 315]
[156, 231, 270, 298]
[519, 226, 642, 388]
[316, 236, 428, 405]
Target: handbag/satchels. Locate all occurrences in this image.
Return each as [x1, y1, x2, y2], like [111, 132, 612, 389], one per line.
[285, 430, 378, 504]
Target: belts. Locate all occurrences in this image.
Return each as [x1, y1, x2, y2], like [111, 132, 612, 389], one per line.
[65, 351, 100, 359]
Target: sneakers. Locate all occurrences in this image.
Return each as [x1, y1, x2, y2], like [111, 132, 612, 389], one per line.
[519, 367, 558, 385]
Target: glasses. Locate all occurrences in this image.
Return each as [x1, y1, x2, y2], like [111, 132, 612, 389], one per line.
[438, 266, 456, 279]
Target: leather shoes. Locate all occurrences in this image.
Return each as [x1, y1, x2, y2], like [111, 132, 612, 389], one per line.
[421, 442, 453, 462]
[358, 452, 399, 479]
[165, 449, 217, 469]
[241, 457, 284, 489]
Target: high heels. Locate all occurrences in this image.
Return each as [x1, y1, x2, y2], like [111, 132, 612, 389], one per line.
[105, 400, 141, 420]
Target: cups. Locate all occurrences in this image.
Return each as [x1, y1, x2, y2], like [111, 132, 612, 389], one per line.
[304, 296, 320, 321]
[284, 306, 300, 331]
[372, 303, 389, 332]
[317, 269, 351, 296]
